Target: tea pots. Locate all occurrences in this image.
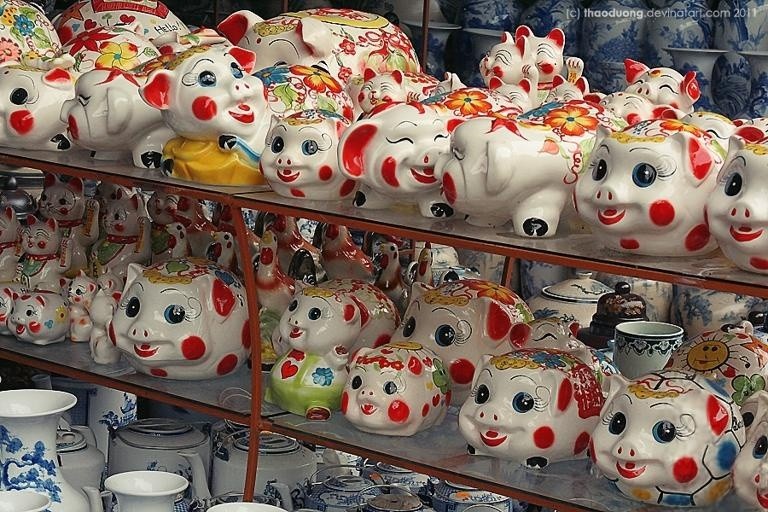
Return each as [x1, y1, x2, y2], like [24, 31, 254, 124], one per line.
[53, 377, 513, 512]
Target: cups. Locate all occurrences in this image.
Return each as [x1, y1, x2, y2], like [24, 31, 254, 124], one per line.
[613, 321, 684, 379]
